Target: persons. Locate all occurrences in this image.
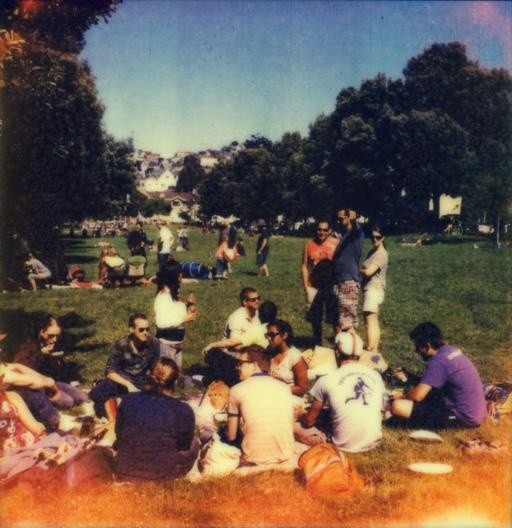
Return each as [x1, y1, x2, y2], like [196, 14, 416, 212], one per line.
[359, 226, 388, 351]
[221, 283, 259, 345]
[152, 259, 197, 373]
[10, 210, 291, 294]
[330, 205, 364, 332]
[300, 218, 341, 340]
[196, 298, 279, 387]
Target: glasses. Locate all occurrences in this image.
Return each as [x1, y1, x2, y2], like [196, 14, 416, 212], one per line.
[138, 327, 151, 333]
[317, 227, 330, 233]
[264, 331, 282, 339]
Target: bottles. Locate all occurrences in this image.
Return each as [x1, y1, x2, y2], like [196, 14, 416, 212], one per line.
[187, 290, 197, 324]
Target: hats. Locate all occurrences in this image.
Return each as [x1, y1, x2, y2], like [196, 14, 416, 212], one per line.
[334, 331, 365, 356]
[256, 218, 268, 226]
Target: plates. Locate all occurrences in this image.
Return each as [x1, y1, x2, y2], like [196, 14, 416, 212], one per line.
[406, 461, 454, 474]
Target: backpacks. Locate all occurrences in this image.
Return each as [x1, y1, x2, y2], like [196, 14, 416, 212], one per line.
[296, 442, 368, 498]
